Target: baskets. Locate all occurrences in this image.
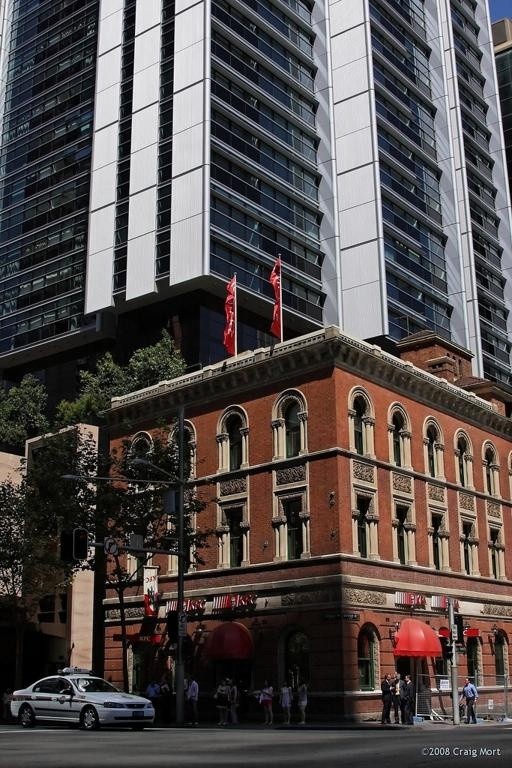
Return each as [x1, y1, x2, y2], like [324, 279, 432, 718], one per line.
[458, 702, 467, 717]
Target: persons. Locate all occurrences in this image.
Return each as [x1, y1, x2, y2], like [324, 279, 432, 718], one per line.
[144, 670, 312, 729]
[459, 677, 480, 724]
[381, 674, 395, 724]
[402, 675, 416, 725]
[392, 672, 405, 724]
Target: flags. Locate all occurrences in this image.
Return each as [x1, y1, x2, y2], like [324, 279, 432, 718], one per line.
[269, 257, 282, 340]
[222, 276, 236, 357]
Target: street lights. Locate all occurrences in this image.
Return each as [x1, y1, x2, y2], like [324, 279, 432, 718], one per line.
[55, 404, 188, 724]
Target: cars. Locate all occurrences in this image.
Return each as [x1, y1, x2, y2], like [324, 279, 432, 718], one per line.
[10, 668, 155, 730]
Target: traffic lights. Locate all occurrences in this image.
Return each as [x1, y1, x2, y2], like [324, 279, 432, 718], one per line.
[74, 526, 87, 560]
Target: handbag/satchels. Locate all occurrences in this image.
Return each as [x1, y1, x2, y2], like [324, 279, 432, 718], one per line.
[214, 692, 218, 699]
